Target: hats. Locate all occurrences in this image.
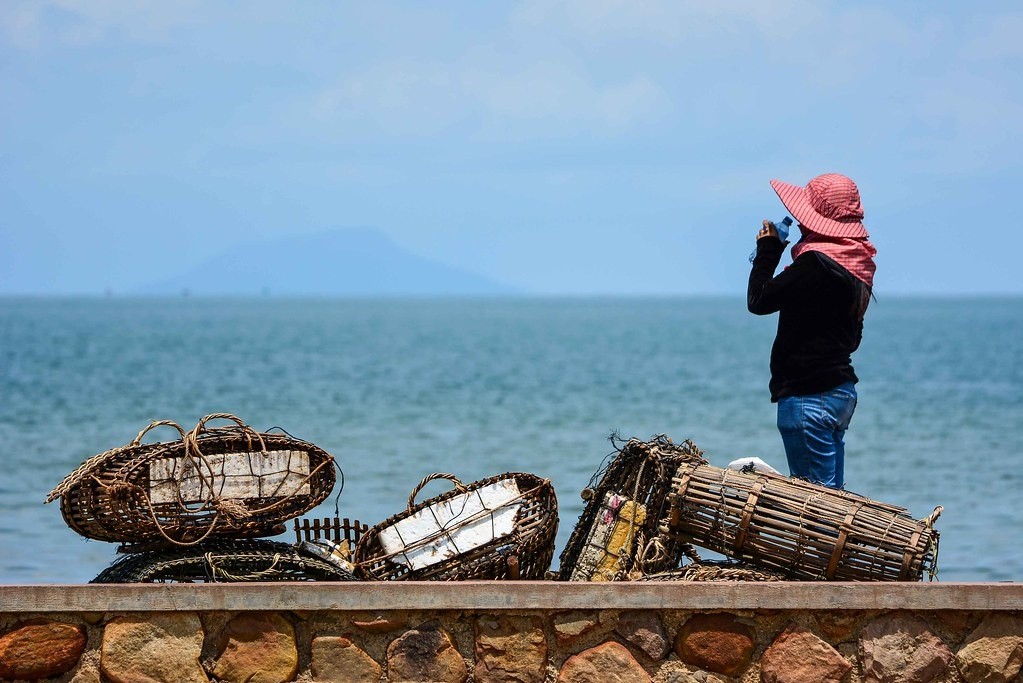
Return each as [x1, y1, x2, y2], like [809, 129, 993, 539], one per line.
[770, 174, 869, 237]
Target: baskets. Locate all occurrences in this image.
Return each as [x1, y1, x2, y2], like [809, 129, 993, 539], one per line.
[44, 413, 336, 542]
[353, 472, 559, 581]
[91, 539, 355, 583]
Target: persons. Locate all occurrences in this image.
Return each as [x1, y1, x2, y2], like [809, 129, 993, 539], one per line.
[747, 174, 878, 555]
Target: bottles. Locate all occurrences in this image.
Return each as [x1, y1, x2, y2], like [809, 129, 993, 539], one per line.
[749, 216, 793, 264]
